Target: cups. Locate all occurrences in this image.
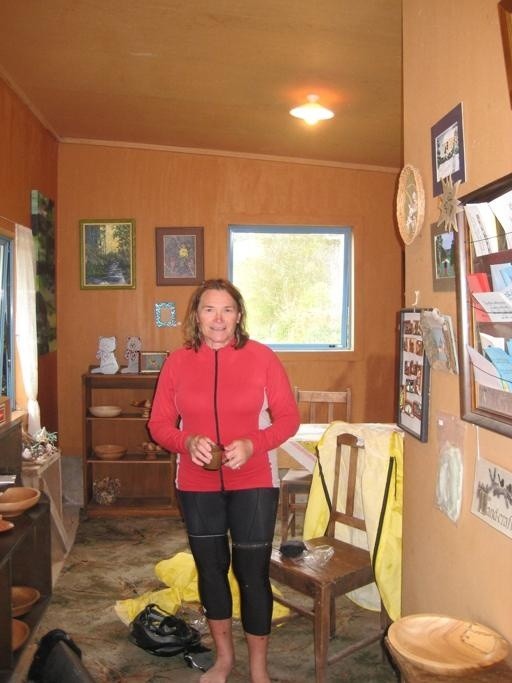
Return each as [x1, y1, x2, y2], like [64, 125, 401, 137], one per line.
[204, 444, 225, 470]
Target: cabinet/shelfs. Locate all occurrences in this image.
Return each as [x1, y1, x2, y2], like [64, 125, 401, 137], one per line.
[82, 362, 187, 520]
[0, 410, 54, 683]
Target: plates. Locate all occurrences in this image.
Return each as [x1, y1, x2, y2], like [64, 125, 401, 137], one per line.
[12, 618, 31, 652]
[395, 162, 425, 244]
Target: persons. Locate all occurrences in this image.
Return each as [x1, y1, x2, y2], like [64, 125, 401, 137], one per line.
[146, 279, 300, 683]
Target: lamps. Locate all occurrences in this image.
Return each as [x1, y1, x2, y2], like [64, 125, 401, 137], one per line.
[286, 94, 340, 127]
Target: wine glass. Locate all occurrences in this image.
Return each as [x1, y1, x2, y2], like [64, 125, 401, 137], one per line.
[137, 444, 162, 460]
[127, 402, 152, 417]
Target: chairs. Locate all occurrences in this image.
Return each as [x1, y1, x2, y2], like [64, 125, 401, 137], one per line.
[279, 384, 354, 549]
[262, 420, 402, 682]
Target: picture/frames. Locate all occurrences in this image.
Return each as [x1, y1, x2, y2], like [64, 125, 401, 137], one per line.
[75, 214, 139, 293]
[152, 224, 207, 288]
[396, 302, 433, 443]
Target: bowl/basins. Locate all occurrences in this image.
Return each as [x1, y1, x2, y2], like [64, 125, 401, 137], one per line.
[90, 405, 121, 417]
[95, 444, 128, 460]
[1, 486, 41, 517]
[11, 585, 41, 618]
